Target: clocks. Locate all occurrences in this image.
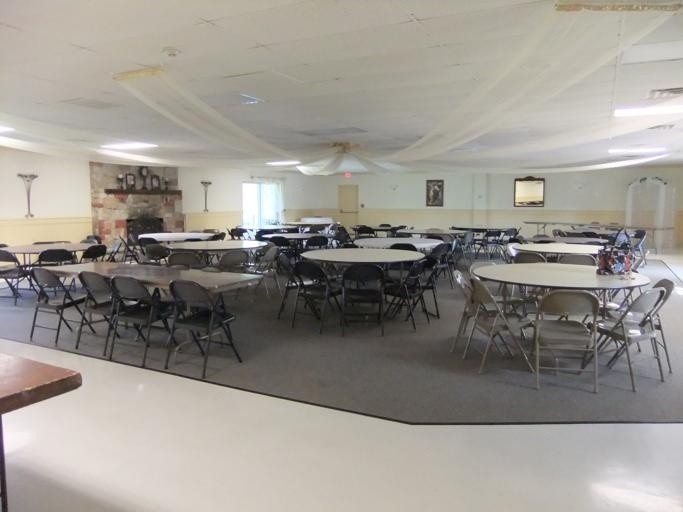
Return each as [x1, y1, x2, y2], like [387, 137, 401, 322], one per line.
[140, 166, 149, 177]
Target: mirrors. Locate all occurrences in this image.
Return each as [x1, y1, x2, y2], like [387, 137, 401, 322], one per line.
[513, 176, 545, 207]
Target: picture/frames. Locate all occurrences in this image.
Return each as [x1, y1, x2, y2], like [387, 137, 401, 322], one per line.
[151, 175, 161, 190]
[426, 179, 443, 206]
[125, 172, 136, 190]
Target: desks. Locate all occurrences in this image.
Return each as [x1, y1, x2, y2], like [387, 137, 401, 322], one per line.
[0, 352, 82, 512]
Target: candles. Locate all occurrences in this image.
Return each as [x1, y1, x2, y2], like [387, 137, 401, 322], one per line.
[165, 177, 168, 182]
[117, 173, 123, 178]
